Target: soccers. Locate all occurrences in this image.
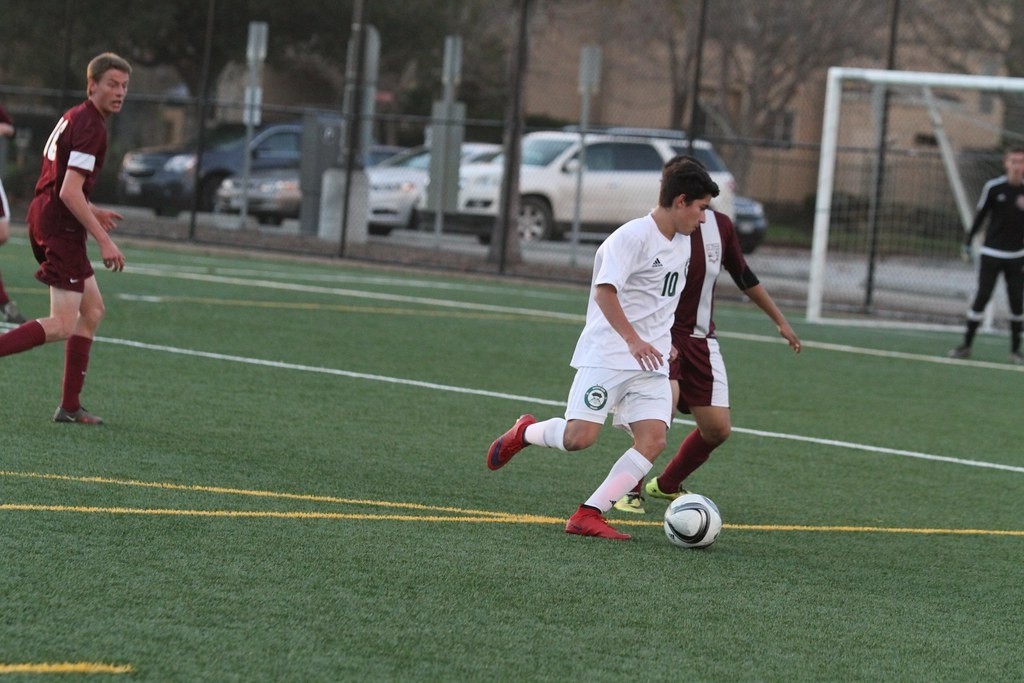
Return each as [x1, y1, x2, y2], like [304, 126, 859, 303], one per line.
[664, 494, 722, 548]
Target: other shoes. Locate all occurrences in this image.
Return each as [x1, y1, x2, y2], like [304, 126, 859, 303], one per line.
[946, 344, 970, 358]
[1009, 352, 1024, 364]
[0, 300, 27, 323]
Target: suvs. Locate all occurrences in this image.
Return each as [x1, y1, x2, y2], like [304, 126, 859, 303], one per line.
[414, 124, 737, 248]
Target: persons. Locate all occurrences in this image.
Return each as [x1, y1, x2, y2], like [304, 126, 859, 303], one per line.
[0, 53, 132, 426]
[611, 156, 803, 515]
[949, 145, 1024, 360]
[486, 152, 720, 538]
[0, 106, 25, 325]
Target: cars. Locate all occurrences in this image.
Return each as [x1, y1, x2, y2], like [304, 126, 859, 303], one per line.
[734, 191, 767, 255]
[116, 114, 548, 238]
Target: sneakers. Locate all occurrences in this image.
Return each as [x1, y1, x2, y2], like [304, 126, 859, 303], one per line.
[53, 403, 103, 424]
[564, 504, 632, 539]
[645, 476, 692, 500]
[613, 493, 646, 514]
[487, 413, 536, 470]
